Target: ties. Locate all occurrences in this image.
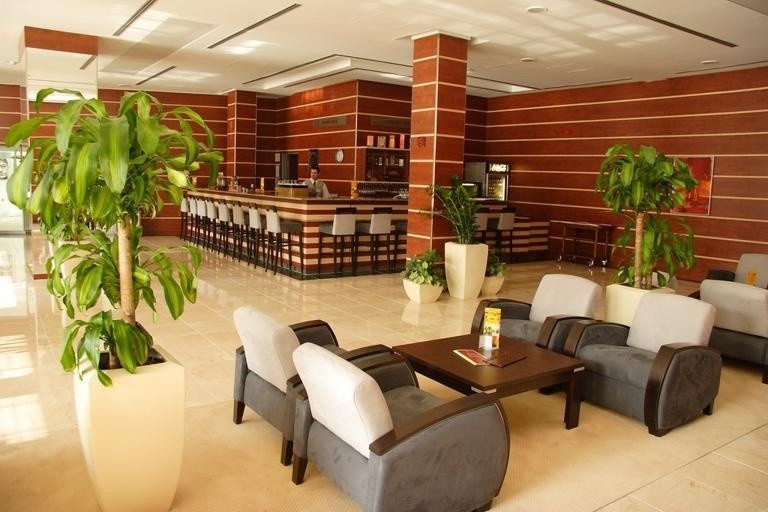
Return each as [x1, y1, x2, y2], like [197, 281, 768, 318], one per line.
[313, 182, 316, 186]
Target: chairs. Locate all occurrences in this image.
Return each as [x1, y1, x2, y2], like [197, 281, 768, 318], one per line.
[356, 207, 393, 274]
[563, 292, 721, 438]
[392, 222, 407, 268]
[291, 342, 511, 510]
[318, 208, 372, 278]
[687, 280, 764, 385]
[705, 253, 768, 289]
[181, 193, 309, 276]
[232, 302, 392, 465]
[489, 209, 517, 264]
[474, 208, 489, 244]
[472, 274, 601, 394]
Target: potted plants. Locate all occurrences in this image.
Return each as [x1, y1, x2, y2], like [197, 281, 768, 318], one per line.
[416, 176, 489, 299]
[400, 247, 447, 303]
[593, 143, 695, 322]
[481, 249, 505, 296]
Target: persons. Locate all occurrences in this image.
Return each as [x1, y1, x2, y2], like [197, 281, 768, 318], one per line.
[0, 159, 9, 180]
[302, 166, 331, 198]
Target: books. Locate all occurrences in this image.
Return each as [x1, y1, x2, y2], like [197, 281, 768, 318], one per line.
[452, 349, 491, 367]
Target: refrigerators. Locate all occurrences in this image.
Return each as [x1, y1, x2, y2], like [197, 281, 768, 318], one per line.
[465, 161, 510, 202]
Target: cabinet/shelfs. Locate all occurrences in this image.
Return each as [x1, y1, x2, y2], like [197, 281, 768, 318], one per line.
[353, 145, 409, 200]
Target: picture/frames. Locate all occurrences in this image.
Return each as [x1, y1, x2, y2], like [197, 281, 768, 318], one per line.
[308, 149, 317, 168]
[334, 150, 344, 163]
